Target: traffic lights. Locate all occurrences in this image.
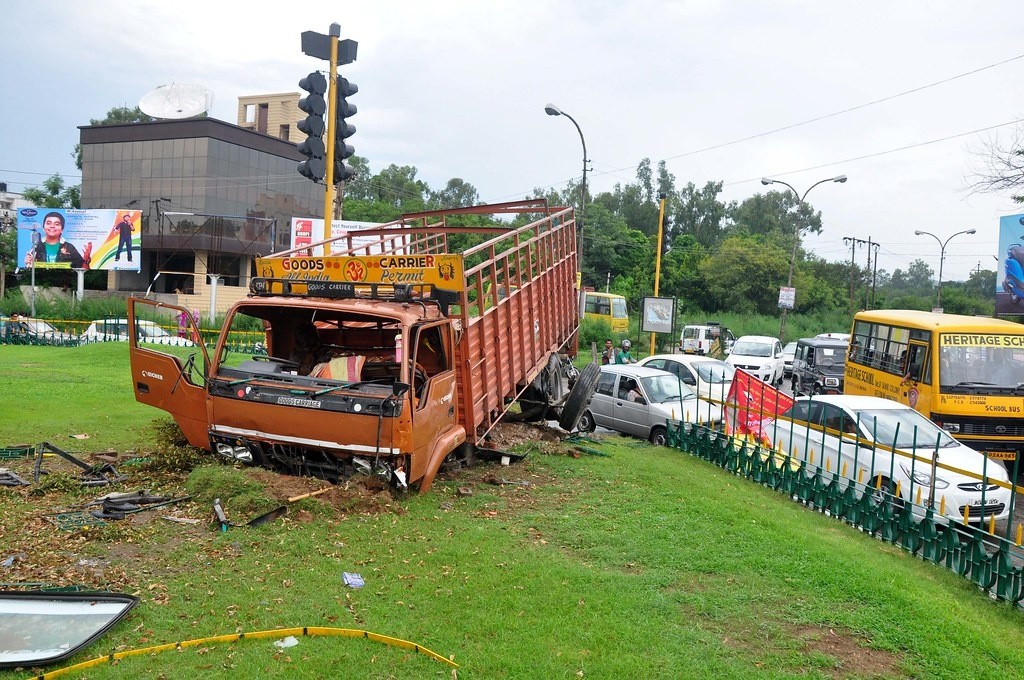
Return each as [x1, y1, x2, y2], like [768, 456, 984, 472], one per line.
[332, 77, 358, 184]
[297, 73, 327, 181]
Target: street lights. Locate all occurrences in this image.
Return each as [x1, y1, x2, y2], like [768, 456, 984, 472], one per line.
[545, 103, 586, 289]
[915, 229, 976, 308]
[761, 175, 848, 343]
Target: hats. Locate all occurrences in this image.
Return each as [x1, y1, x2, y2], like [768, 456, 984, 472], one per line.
[123, 214, 130, 218]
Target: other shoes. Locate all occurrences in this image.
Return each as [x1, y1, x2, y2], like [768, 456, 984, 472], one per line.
[128, 260, 132, 262]
[115, 258, 120, 261]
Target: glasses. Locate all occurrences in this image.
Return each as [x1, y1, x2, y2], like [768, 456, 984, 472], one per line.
[13, 316, 18, 317]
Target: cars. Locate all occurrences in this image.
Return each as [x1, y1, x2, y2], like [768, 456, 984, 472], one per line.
[725, 335, 784, 389]
[626, 353, 734, 411]
[79, 319, 196, 347]
[577, 364, 723, 448]
[783, 342, 798, 377]
[764, 395, 1015, 526]
[0, 318, 78, 344]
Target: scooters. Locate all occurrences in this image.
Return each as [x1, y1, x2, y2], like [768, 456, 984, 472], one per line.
[1002, 243, 1024, 303]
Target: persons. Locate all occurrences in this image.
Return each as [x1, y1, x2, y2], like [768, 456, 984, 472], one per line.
[25, 212, 93, 269]
[899, 364, 929, 386]
[847, 420, 859, 434]
[628, 381, 644, 403]
[616, 340, 637, 364]
[175, 311, 187, 338]
[601, 339, 619, 365]
[190, 309, 199, 343]
[114, 215, 135, 262]
[5, 313, 29, 345]
[849, 341, 862, 361]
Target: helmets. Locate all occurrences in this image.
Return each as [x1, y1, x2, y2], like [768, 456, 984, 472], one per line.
[712, 332, 718, 337]
[622, 340, 631, 348]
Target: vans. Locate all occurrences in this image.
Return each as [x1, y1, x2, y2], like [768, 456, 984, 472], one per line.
[679, 322, 736, 356]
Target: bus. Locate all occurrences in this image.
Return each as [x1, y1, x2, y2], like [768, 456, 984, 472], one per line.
[843, 309, 1024, 480]
[484, 283, 630, 334]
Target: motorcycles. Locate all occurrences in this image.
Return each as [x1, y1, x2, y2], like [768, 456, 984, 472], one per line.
[559, 355, 579, 381]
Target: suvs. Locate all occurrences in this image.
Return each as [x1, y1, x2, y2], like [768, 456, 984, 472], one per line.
[789, 337, 849, 398]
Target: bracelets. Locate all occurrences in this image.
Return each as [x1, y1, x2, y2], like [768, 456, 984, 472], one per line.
[83, 257, 91, 264]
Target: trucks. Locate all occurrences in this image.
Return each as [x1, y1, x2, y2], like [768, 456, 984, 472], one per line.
[126, 198, 604, 497]
